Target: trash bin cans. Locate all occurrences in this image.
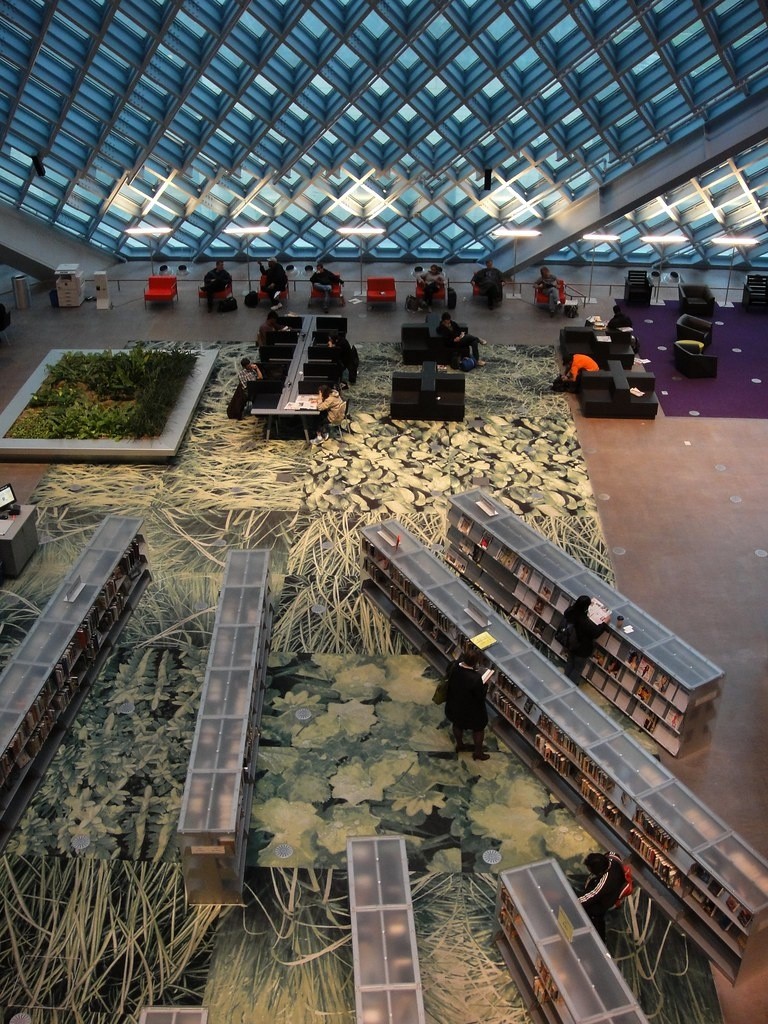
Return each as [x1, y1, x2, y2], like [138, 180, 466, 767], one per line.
[11, 274, 31, 309]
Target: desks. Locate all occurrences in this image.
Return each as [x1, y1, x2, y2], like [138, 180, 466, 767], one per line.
[0, 506, 40, 578]
[251, 314, 347, 445]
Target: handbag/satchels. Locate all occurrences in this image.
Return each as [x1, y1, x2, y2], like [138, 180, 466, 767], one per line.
[554, 621, 577, 647]
[431, 680, 449, 706]
[244, 290, 258, 307]
[550, 374, 577, 393]
[458, 356, 476, 371]
[217, 297, 238, 312]
[564, 304, 578, 318]
[405, 295, 425, 313]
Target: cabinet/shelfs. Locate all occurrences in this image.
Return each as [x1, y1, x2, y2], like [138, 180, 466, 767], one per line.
[176, 547, 274, 909]
[490, 856, 652, 1024]
[359, 516, 768, 987]
[446, 490, 725, 759]
[347, 835, 425, 1024]
[0, 514, 156, 853]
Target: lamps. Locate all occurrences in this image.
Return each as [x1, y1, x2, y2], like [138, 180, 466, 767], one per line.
[123, 227, 174, 277]
[583, 233, 758, 307]
[492, 229, 541, 299]
[336, 227, 387, 296]
[224, 227, 269, 292]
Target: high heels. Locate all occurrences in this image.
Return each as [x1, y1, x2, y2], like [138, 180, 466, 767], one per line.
[472, 751, 489, 762]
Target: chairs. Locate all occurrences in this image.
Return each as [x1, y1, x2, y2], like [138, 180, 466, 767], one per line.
[143, 276, 179, 311]
[0, 312, 11, 347]
[416, 275, 446, 307]
[365, 276, 396, 310]
[322, 401, 351, 444]
[472, 272, 479, 303]
[237, 372, 251, 419]
[258, 273, 289, 303]
[535, 280, 565, 309]
[673, 284, 718, 379]
[199, 274, 232, 307]
[742, 273, 768, 314]
[308, 273, 344, 307]
[623, 268, 654, 304]
[346, 345, 359, 383]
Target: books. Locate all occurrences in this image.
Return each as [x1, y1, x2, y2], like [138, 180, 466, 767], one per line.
[450, 512, 692, 762]
[0, 537, 143, 779]
[354, 535, 767, 1004]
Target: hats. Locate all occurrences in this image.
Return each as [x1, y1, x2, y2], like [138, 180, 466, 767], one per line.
[266, 257, 278, 263]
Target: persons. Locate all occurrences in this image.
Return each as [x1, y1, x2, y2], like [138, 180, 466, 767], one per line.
[309, 263, 345, 313]
[561, 353, 600, 393]
[199, 259, 231, 313]
[562, 596, 610, 686]
[257, 258, 288, 310]
[532, 266, 566, 317]
[607, 305, 633, 329]
[311, 384, 344, 445]
[578, 851, 633, 946]
[436, 312, 487, 366]
[327, 332, 351, 370]
[238, 358, 263, 397]
[470, 259, 505, 310]
[417, 264, 448, 313]
[259, 311, 278, 346]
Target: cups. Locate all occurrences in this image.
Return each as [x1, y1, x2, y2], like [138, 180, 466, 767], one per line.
[617, 616, 624, 628]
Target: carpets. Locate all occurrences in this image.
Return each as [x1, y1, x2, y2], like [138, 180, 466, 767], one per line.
[613, 298, 768, 419]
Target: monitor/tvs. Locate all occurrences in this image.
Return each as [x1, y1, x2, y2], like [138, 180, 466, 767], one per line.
[0, 483, 17, 511]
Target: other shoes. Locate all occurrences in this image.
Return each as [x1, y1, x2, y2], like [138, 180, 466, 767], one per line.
[207, 307, 212, 313]
[273, 291, 281, 299]
[478, 337, 487, 346]
[323, 427, 330, 442]
[550, 312, 554, 317]
[200, 287, 206, 292]
[427, 307, 433, 312]
[474, 361, 486, 367]
[271, 302, 282, 311]
[310, 438, 325, 445]
[324, 306, 329, 314]
[557, 302, 561, 308]
[487, 303, 492, 310]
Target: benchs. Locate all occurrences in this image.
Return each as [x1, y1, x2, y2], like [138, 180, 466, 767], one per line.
[558, 315, 660, 420]
[391, 312, 468, 423]
